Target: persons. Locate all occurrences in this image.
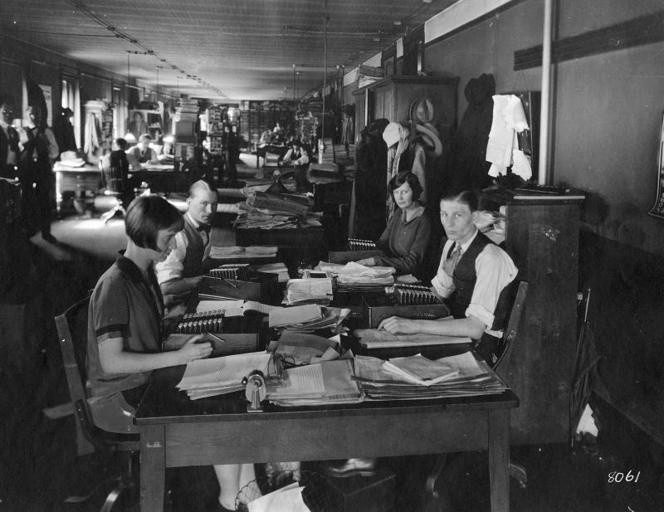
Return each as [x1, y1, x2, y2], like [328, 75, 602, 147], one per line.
[107, 136, 140, 216]
[129, 131, 161, 194]
[1, 96, 31, 299]
[284, 142, 307, 162]
[82, 192, 266, 511]
[152, 180, 221, 335]
[350, 169, 432, 278]
[128, 112, 147, 140]
[18, 99, 59, 244]
[316, 186, 521, 481]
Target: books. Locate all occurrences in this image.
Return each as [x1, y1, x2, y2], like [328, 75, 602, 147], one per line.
[179, 240, 512, 407]
[504, 188, 588, 201]
[232, 185, 322, 233]
[319, 139, 359, 166]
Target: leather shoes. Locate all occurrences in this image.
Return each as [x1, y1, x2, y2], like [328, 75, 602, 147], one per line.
[328, 459, 375, 479]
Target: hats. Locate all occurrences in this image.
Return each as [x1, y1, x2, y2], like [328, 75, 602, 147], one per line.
[464, 73, 489, 106]
[382, 121, 411, 148]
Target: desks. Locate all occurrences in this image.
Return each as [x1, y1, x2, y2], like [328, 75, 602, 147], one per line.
[55, 162, 188, 220]
[133, 243, 519, 510]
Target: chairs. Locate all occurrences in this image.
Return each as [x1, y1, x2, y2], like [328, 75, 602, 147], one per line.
[423, 281, 527, 500]
[40, 289, 139, 511]
[97, 158, 141, 223]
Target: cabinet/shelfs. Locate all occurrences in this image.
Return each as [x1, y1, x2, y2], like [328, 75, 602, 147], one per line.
[476, 193, 581, 448]
